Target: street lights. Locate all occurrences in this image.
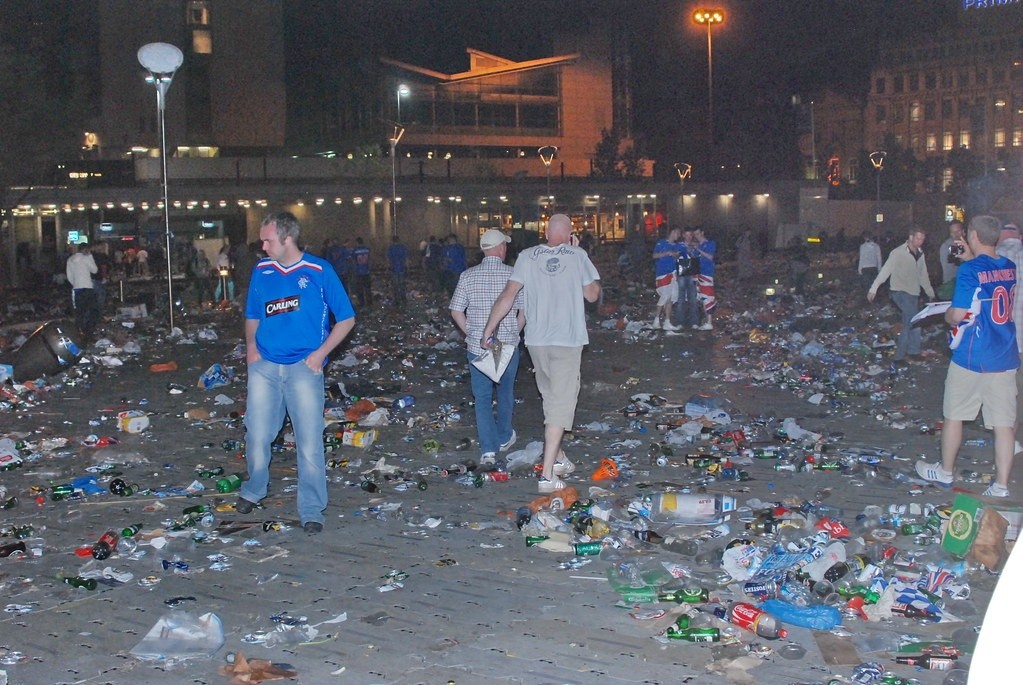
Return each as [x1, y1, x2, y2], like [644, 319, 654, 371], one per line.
[384, 118, 405, 238]
[389, 84, 409, 244]
[537, 145, 557, 218]
[869, 151, 887, 240]
[692, 7, 724, 238]
[674, 162, 691, 229]
[137, 42, 185, 333]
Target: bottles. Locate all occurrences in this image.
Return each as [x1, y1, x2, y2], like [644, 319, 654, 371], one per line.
[0, 300, 1023, 685]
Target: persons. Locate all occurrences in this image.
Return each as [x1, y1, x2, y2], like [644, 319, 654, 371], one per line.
[867, 223, 937, 367]
[915, 215, 1021, 497]
[66, 242, 98, 333]
[992, 223, 1023, 470]
[449, 230, 526, 465]
[235, 210, 356, 533]
[480, 213, 601, 494]
[0, 223, 1023, 367]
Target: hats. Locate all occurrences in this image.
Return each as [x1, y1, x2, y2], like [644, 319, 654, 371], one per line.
[480, 230, 512, 250]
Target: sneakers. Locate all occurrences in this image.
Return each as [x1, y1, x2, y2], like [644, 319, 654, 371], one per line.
[236, 497, 254, 513]
[537, 474, 566, 492]
[915, 460, 953, 484]
[553, 451, 575, 476]
[480, 452, 495, 465]
[304, 522, 323, 533]
[499, 429, 516, 452]
[983, 483, 1009, 497]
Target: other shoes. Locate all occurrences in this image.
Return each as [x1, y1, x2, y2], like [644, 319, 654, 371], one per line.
[699, 323, 713, 330]
[910, 353, 925, 361]
[896, 360, 910, 368]
[652, 319, 661, 329]
[663, 322, 678, 330]
[677, 325, 684, 330]
[692, 325, 699, 329]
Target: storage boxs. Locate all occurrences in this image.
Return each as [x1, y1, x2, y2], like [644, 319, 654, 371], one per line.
[987, 502, 1023, 540]
[942, 493, 1008, 573]
